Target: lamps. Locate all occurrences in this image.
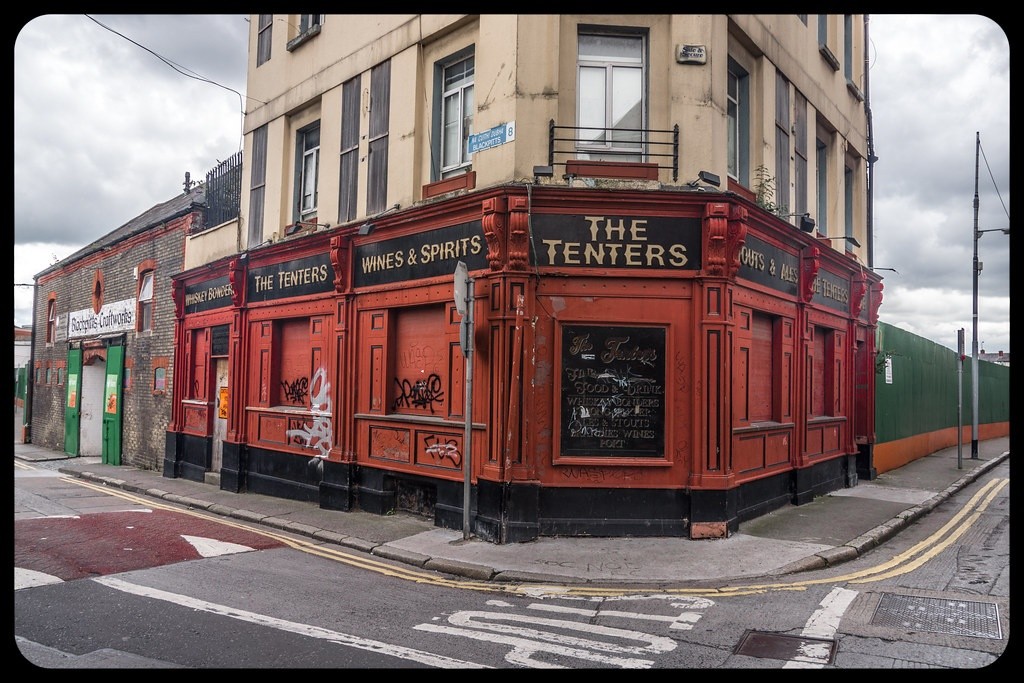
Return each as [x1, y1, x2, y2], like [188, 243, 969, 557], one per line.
[357, 203, 401, 236]
[816, 236, 861, 248]
[240, 239, 274, 265]
[287, 222, 331, 235]
[774, 213, 816, 233]
[533, 165, 554, 185]
[687, 170, 721, 187]
